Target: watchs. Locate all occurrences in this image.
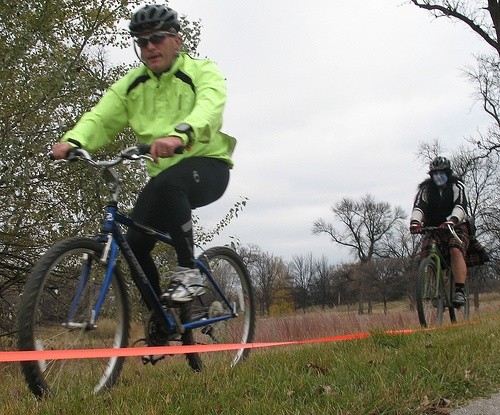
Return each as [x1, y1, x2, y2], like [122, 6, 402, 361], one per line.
[174, 123, 194, 145]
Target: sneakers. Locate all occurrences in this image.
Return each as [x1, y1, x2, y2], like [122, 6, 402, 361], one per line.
[452, 292, 467, 305]
[160, 265, 206, 308]
[144, 335, 184, 361]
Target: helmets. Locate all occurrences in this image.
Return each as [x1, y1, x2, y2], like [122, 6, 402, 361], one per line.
[128, 4, 181, 40]
[429, 157, 450, 170]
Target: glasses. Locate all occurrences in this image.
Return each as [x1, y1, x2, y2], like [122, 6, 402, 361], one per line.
[138, 33, 175, 48]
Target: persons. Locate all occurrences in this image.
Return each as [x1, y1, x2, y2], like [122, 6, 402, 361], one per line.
[410, 156, 469, 305]
[51, 4, 238, 364]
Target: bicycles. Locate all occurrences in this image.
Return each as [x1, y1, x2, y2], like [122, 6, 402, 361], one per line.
[412, 224, 469, 329]
[16, 144, 257, 402]
